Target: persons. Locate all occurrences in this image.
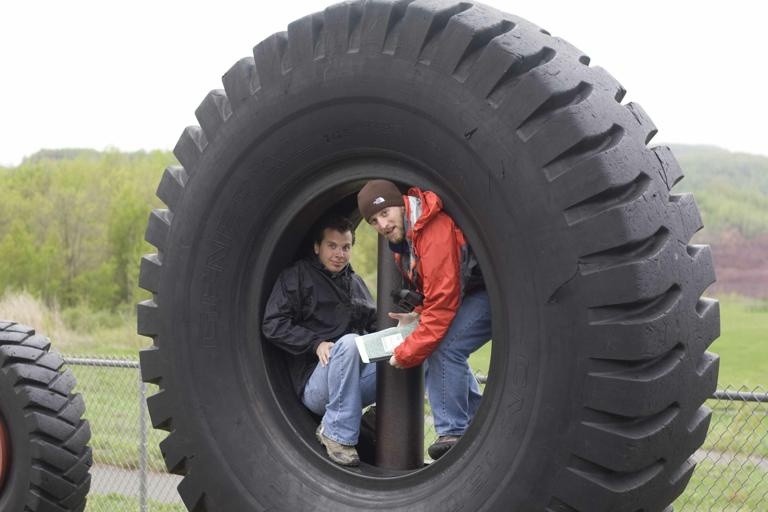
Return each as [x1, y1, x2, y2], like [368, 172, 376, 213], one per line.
[259, 212, 430, 468]
[356, 178, 492, 465]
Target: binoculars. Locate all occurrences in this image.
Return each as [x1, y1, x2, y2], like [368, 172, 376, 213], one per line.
[389, 288, 424, 313]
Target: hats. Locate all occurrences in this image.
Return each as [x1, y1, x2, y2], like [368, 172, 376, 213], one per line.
[357, 179, 405, 222]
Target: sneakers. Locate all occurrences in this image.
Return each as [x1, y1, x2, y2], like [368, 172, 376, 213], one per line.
[360, 407, 378, 434]
[428, 435, 459, 460]
[315, 424, 360, 466]
